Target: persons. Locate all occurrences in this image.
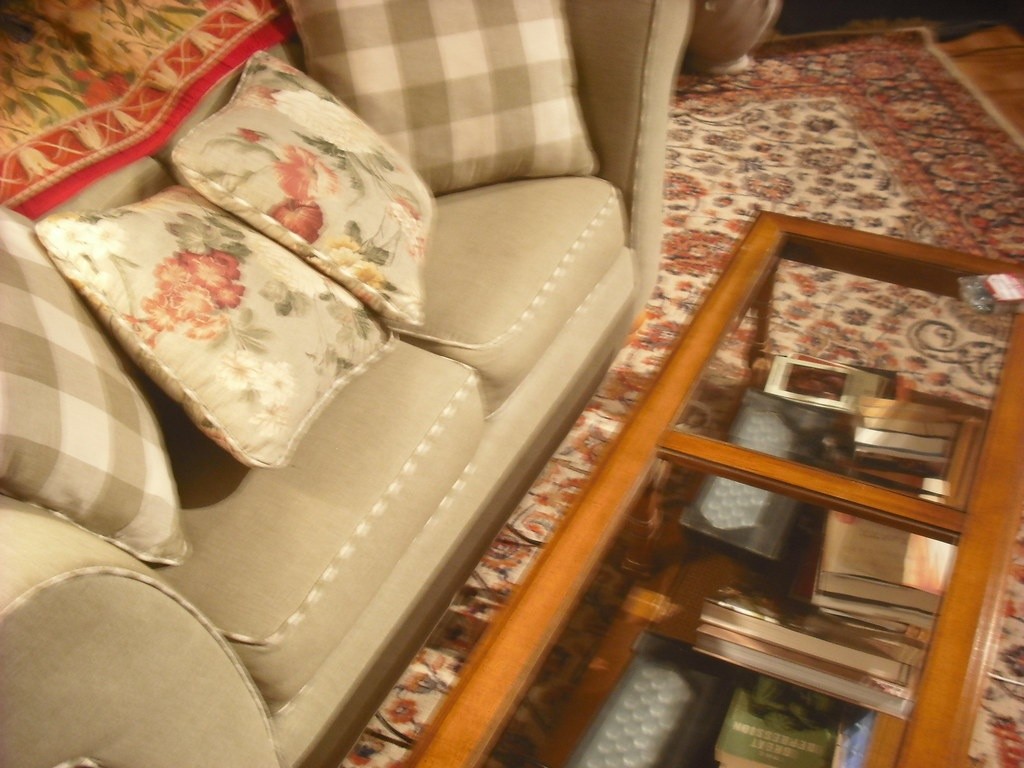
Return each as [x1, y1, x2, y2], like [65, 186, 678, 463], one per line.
[795, 368, 845, 401]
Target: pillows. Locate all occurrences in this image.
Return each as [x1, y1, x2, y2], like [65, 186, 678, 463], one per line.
[0, 206, 187, 561]
[172, 49, 435, 330]
[35, 187, 394, 474]
[287, 0, 599, 194]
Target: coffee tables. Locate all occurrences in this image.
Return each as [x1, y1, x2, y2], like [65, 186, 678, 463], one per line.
[394, 211, 1024, 768]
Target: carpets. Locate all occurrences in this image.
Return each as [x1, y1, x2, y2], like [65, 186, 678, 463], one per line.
[336, 21, 1024, 768]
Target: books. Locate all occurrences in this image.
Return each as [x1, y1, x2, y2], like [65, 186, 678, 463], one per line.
[692, 397, 960, 768]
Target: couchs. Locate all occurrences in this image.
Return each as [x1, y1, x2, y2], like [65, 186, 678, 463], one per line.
[0, 0, 694, 768]
